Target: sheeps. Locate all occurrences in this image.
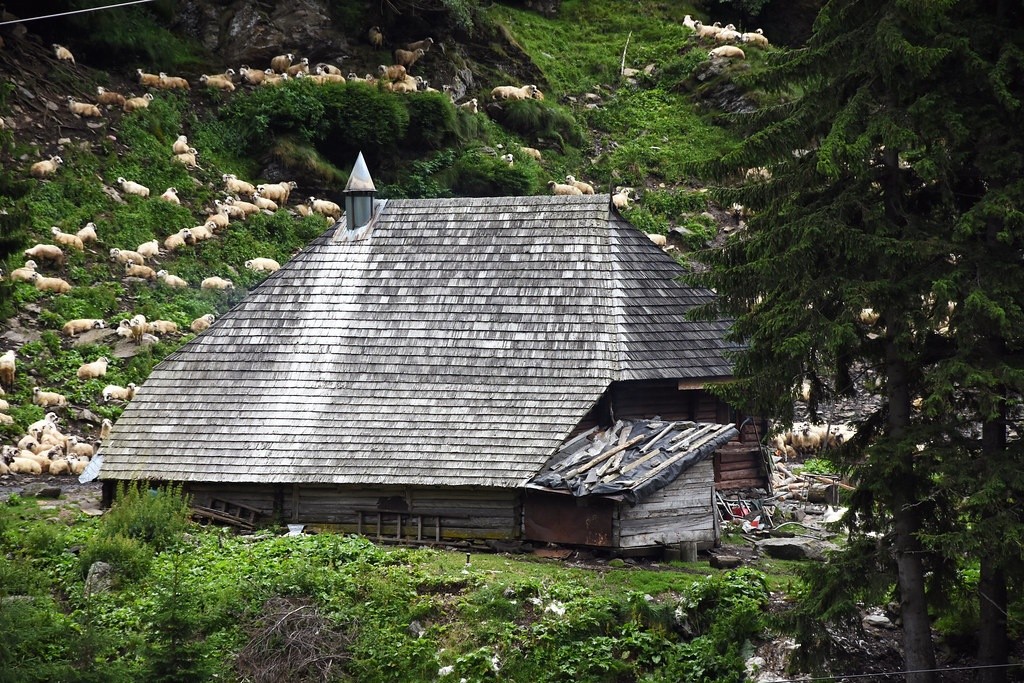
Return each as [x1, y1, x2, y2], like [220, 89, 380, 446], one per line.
[515, 143, 542, 160]
[29, 25, 435, 258]
[613, 166, 773, 249]
[548, 181, 582, 195]
[684, 14, 768, 59]
[791, 300, 958, 403]
[489, 85, 544, 100]
[111, 248, 144, 265]
[50, 226, 85, 250]
[0, 244, 177, 476]
[500, 154, 514, 166]
[157, 271, 189, 286]
[771, 421, 858, 461]
[125, 259, 156, 277]
[246, 258, 281, 272]
[461, 98, 479, 114]
[566, 176, 594, 194]
[78, 222, 98, 242]
[201, 277, 233, 290]
[191, 314, 215, 334]
